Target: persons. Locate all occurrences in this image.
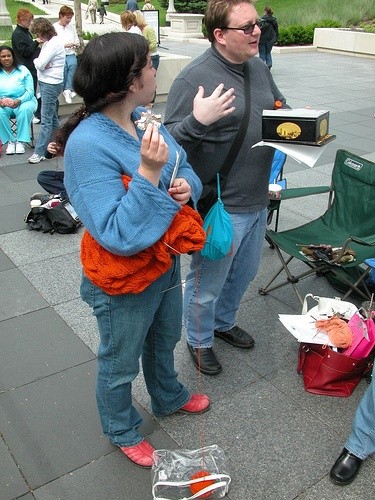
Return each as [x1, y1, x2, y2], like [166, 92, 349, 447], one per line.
[142, 0, 155, 10]
[163, 0, 310, 376]
[97, 4, 107, 24]
[259, 7, 279, 70]
[37, 141, 66, 195]
[329, 356, 375, 487]
[54, 32, 211, 469]
[32, 0, 49, 4]
[53, 6, 80, 103]
[133, 10, 159, 109]
[85, 0, 98, 24]
[126, 0, 140, 12]
[28, 17, 66, 164]
[11, 8, 43, 124]
[120, 10, 143, 36]
[0, 46, 38, 154]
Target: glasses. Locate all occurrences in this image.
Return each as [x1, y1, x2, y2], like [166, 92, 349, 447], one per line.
[223, 22, 264, 34]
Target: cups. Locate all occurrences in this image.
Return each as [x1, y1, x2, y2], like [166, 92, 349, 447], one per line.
[268, 183, 282, 199]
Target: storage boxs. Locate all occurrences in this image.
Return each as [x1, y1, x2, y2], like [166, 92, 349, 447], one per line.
[262, 108, 330, 142]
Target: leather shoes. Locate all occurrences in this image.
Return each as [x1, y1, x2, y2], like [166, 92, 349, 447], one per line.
[329, 448, 362, 486]
[187, 342, 222, 375]
[176, 393, 211, 416]
[119, 438, 159, 469]
[214, 325, 255, 349]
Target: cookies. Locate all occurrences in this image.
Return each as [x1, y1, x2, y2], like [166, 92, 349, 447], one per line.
[135, 110, 162, 130]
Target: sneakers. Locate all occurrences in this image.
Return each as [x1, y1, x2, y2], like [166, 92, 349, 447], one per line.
[33, 117, 40, 123]
[28, 153, 46, 164]
[6, 141, 15, 154]
[16, 141, 25, 154]
[62, 89, 76, 104]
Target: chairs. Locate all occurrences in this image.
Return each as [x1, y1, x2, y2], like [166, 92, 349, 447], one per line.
[266, 149, 288, 249]
[9, 115, 35, 149]
[258, 149, 375, 315]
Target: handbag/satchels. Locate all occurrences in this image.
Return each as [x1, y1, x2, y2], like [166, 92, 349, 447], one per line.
[24, 192, 82, 234]
[200, 173, 234, 261]
[297, 293, 375, 398]
